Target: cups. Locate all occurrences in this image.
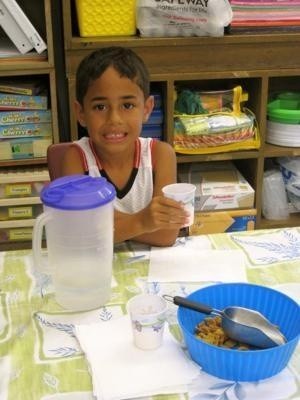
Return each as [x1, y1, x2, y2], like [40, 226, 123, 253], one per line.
[160, 182, 197, 228]
[125, 292, 168, 353]
[30, 174, 117, 313]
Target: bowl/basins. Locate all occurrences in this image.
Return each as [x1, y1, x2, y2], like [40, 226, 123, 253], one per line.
[176, 282, 300, 384]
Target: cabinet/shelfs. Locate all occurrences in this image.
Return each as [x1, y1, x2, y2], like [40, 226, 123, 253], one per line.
[0, 1, 300, 252]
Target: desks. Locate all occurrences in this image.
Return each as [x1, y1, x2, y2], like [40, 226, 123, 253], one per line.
[0, 225, 299, 398]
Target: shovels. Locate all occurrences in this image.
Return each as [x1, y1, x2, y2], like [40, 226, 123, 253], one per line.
[163, 295, 288, 349]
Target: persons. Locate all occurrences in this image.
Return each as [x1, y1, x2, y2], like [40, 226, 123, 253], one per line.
[58, 46, 180, 249]
